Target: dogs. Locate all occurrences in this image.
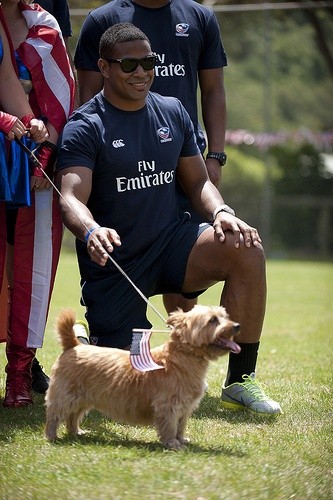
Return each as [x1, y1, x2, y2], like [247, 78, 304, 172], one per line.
[43, 303, 242, 453]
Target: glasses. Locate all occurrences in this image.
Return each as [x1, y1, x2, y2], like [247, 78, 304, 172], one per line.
[105, 54, 156, 73]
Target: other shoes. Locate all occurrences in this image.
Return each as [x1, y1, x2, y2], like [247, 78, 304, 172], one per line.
[31, 357, 49, 392]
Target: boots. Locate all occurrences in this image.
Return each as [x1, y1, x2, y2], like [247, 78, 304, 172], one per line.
[3, 342, 34, 407]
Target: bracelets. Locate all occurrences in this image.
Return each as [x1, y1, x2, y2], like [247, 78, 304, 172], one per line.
[84, 225, 99, 242]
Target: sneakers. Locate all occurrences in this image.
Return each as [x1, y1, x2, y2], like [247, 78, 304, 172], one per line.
[222, 372, 282, 417]
[73, 320, 89, 342]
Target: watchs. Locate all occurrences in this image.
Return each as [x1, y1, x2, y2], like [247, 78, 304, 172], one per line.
[206, 151, 228, 168]
[212, 204, 236, 222]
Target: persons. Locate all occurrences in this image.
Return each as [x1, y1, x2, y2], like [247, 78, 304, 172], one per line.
[1, 1, 79, 408]
[74, 0, 230, 328]
[58, 24, 284, 416]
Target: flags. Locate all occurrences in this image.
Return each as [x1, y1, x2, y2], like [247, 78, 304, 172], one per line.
[128, 329, 165, 373]
